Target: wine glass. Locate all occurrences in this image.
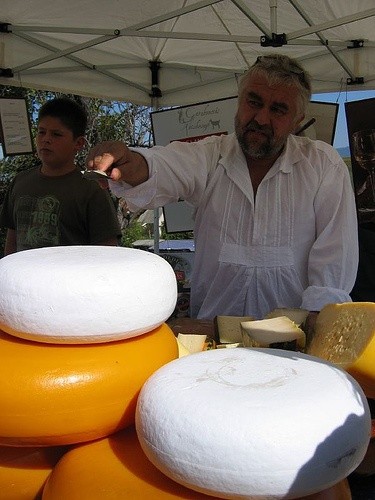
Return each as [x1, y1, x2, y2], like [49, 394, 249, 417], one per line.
[352, 129, 375, 200]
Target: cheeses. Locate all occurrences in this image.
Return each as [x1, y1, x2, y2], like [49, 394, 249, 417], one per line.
[0, 245, 375, 500]
